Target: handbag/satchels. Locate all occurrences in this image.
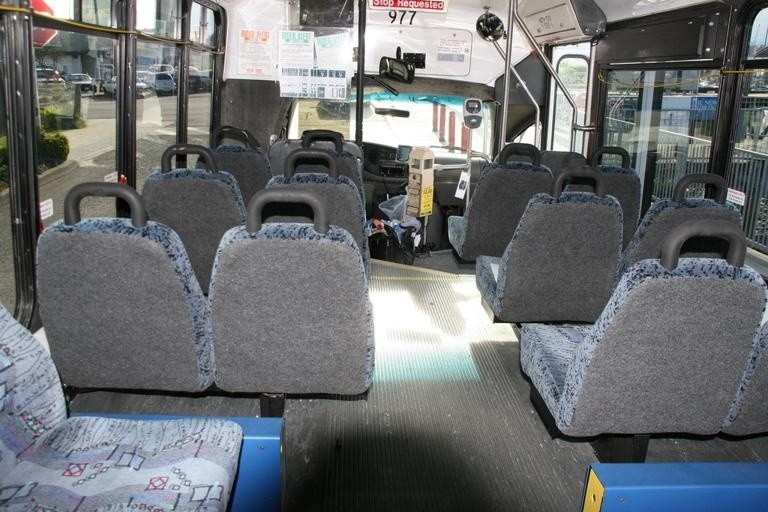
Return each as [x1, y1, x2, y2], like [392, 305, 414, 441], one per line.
[390, 222, 418, 265]
[368, 220, 401, 263]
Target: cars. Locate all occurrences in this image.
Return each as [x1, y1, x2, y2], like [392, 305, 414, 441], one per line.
[30, 63, 212, 99]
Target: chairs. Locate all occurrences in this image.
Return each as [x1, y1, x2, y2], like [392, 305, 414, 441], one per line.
[1, 128, 376, 509]
[447, 144, 768, 512]
[220, 79, 293, 148]
